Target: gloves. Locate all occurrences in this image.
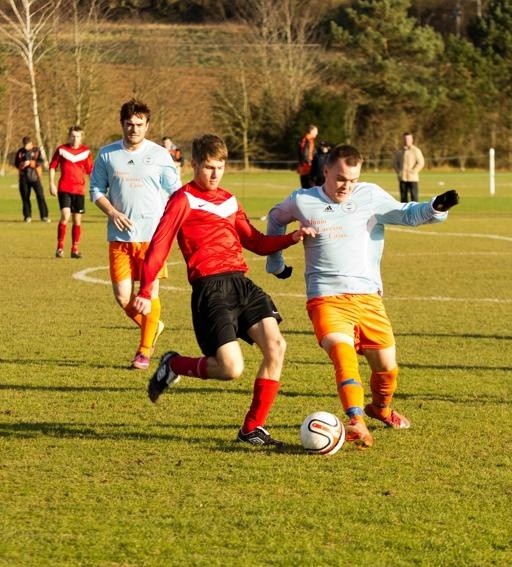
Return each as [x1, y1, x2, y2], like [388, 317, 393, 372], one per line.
[273, 265, 293, 279]
[432, 189, 460, 212]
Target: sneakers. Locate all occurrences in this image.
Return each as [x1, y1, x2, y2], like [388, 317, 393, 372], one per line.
[363, 404, 410, 430]
[56, 249, 64, 257]
[132, 345, 151, 369]
[25, 217, 32, 223]
[344, 420, 374, 451]
[150, 319, 164, 357]
[42, 217, 51, 222]
[147, 351, 181, 404]
[70, 252, 82, 258]
[236, 426, 286, 449]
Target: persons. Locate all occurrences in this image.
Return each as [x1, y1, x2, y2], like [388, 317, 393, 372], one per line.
[13, 136, 50, 224]
[309, 142, 331, 185]
[88, 102, 183, 373]
[392, 132, 425, 202]
[298, 124, 319, 189]
[49, 127, 94, 258]
[266, 148, 459, 446]
[160, 137, 184, 174]
[132, 134, 320, 454]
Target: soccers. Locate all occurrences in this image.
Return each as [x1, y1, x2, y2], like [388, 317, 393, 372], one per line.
[301, 411, 346, 455]
